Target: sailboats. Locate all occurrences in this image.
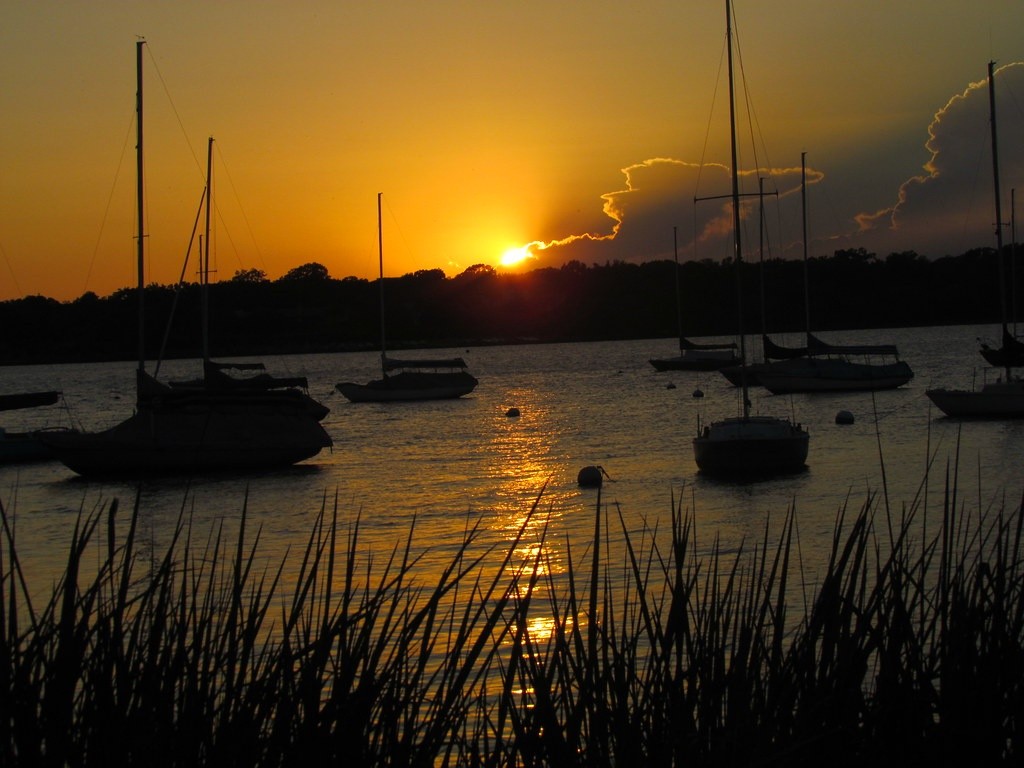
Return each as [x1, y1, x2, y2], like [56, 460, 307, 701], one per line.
[762, 150, 913, 394]
[978, 189, 1023, 369]
[333, 189, 481, 402]
[693, 0, 810, 385]
[648, 225, 741, 372]
[66, 34, 335, 482]
[167, 134, 332, 423]
[926, 61, 1024, 423]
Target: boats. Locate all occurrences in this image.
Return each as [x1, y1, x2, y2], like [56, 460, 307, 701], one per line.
[0, 389, 94, 463]
[693, 381, 812, 481]
